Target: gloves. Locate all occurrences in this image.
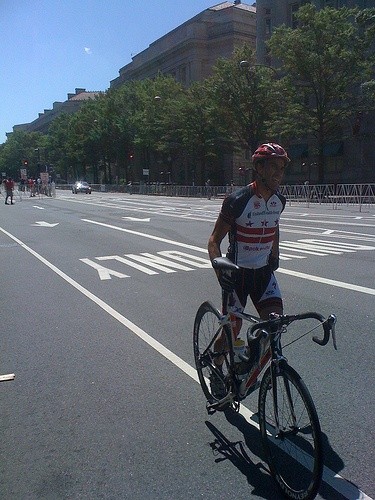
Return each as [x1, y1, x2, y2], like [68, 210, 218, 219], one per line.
[216, 269, 237, 293]
[268, 255, 279, 273]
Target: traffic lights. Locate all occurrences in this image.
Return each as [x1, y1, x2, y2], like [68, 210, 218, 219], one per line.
[24, 160, 28, 167]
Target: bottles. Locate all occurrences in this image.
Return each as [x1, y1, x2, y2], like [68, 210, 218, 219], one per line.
[234, 337, 245, 363]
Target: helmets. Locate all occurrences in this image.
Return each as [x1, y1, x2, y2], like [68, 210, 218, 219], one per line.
[252, 142, 291, 171]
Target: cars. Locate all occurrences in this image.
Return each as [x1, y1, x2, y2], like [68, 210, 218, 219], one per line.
[71, 180, 92, 194]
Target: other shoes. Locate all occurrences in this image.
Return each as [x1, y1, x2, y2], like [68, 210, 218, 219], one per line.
[5, 203, 10, 206]
[209, 372, 226, 399]
[11, 203, 15, 205]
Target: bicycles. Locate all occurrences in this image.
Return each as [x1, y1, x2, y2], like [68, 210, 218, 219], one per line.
[191, 256, 341, 500]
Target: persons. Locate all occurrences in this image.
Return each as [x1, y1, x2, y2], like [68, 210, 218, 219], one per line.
[2, 177, 42, 205]
[209, 144, 290, 399]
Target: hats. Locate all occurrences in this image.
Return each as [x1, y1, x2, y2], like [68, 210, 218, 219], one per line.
[8, 177, 12, 181]
